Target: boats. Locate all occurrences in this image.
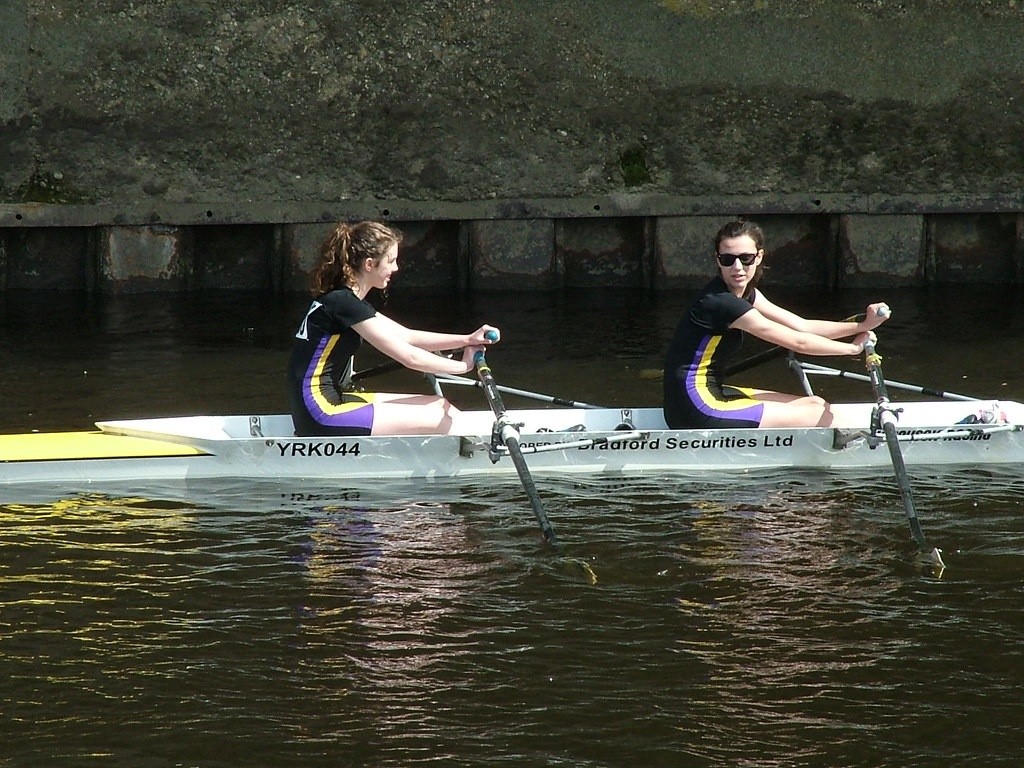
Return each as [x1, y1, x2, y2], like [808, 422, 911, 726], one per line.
[1, 400, 1024, 484]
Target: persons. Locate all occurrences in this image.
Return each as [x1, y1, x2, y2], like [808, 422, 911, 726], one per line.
[285, 222, 501, 436]
[662, 219, 889, 428]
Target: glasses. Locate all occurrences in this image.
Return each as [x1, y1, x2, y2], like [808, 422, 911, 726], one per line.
[716, 249, 759, 267]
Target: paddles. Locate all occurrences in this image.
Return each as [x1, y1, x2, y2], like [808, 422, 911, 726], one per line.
[348, 347, 465, 383]
[864, 340, 945, 579]
[474, 351, 596, 597]
[637, 306, 888, 385]
